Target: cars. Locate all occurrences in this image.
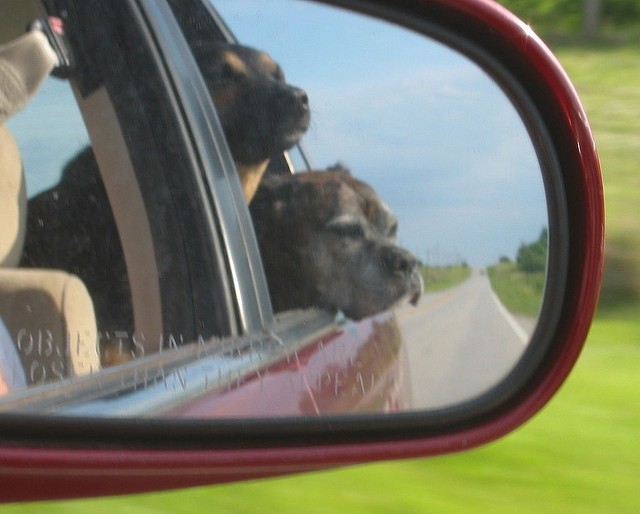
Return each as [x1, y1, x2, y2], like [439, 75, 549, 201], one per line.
[0, 0, 416, 423]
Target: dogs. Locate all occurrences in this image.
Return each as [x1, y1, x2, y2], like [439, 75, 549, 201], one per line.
[17, 40, 319, 371]
[248, 160, 424, 327]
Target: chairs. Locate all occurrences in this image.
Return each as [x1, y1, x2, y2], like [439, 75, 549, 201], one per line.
[0, 124, 99, 387]
